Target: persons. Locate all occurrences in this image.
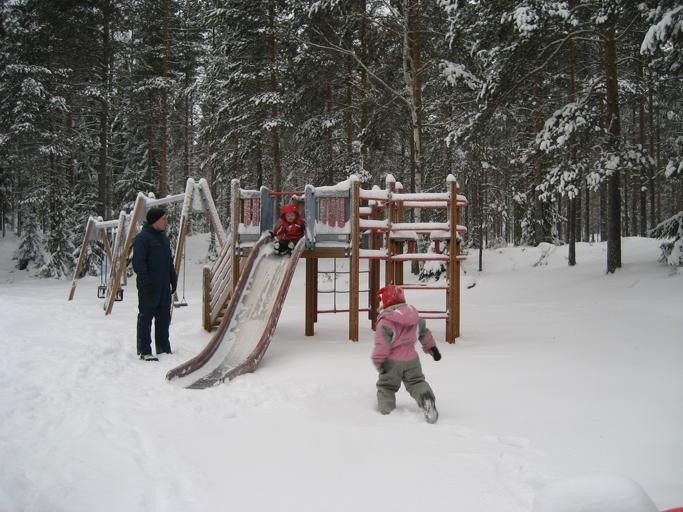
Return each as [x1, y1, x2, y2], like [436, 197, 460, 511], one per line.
[132, 207, 178, 360]
[371, 284, 442, 424]
[268, 204, 306, 257]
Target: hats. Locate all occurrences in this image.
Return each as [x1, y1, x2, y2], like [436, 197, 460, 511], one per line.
[376, 286, 405, 308]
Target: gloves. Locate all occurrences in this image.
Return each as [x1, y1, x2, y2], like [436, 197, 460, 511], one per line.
[431, 347, 441, 361]
[170, 278, 177, 294]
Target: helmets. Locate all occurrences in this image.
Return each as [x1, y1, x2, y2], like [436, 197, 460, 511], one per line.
[146, 208, 165, 224]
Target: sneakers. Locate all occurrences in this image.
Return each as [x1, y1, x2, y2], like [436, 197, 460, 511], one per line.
[422, 395, 438, 423]
[141, 354, 158, 361]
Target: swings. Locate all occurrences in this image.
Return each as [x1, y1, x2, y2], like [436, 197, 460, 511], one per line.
[165, 200, 187, 308]
[97, 225, 124, 301]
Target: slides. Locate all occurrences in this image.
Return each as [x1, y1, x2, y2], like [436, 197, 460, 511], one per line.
[166, 237, 306, 390]
